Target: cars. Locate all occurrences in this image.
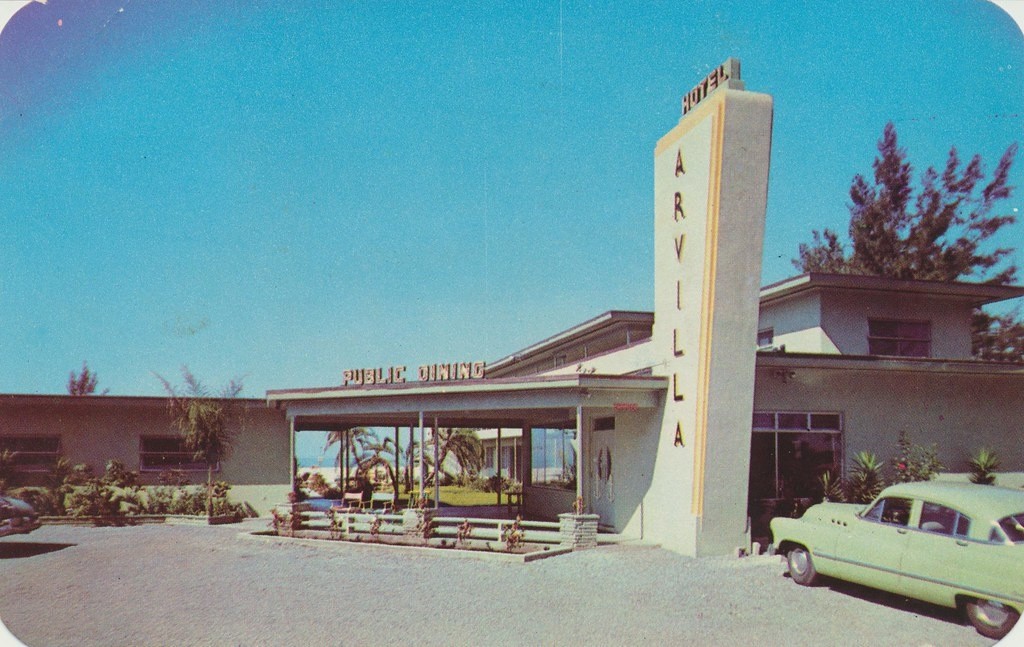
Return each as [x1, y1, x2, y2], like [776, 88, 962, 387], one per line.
[770, 480, 1024, 639]
[0, 495, 41, 537]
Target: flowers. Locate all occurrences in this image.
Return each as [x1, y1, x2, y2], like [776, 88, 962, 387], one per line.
[889, 430, 914, 487]
